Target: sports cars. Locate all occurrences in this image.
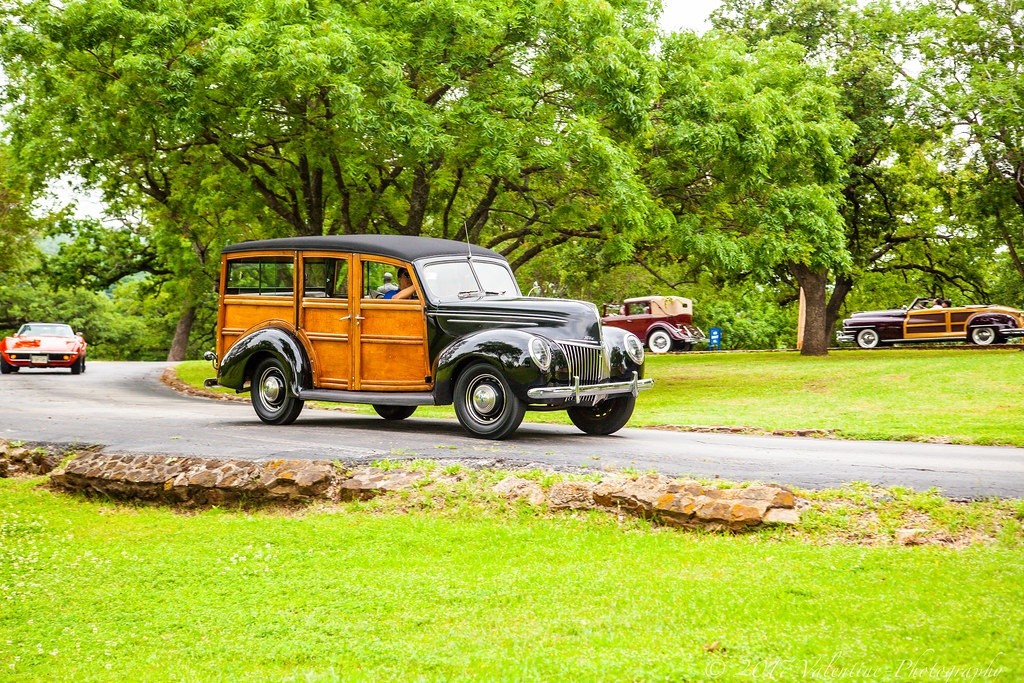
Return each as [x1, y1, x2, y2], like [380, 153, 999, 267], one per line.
[0, 322, 88, 375]
[835, 296, 1024, 350]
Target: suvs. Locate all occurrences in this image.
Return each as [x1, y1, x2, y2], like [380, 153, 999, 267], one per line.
[203, 236, 654, 439]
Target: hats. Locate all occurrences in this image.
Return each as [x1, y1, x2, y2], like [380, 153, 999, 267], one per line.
[383, 272, 392, 280]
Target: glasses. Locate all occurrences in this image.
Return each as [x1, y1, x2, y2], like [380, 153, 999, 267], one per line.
[400, 273, 410, 279]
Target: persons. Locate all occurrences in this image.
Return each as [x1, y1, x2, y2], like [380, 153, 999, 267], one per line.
[377, 271, 397, 293]
[385, 269, 416, 299]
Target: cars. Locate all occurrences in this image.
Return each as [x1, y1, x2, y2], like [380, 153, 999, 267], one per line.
[600, 295, 708, 355]
